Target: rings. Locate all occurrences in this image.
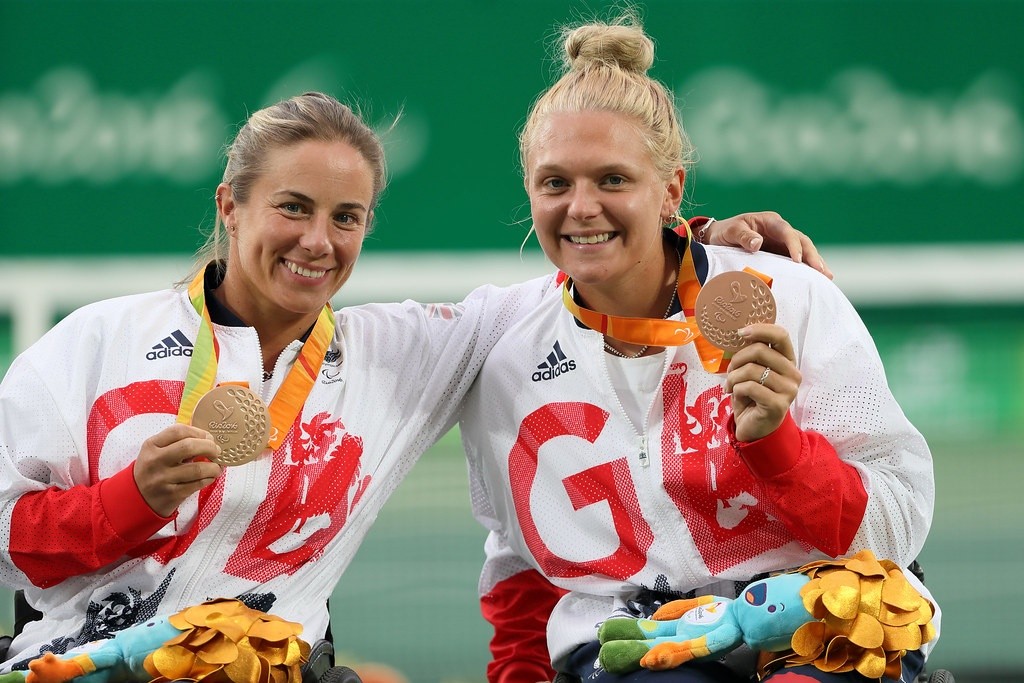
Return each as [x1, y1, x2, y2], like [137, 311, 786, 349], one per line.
[759, 366, 771, 385]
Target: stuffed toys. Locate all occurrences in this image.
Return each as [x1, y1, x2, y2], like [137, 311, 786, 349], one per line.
[596, 551, 938, 681]
[1, 607, 306, 683]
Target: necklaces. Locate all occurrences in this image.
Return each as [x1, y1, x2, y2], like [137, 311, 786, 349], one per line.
[604, 246, 682, 359]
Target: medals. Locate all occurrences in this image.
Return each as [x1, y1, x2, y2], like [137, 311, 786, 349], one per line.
[693, 269, 778, 355]
[185, 382, 272, 467]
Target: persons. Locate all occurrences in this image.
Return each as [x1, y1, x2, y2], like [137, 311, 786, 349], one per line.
[461, 17, 943, 683]
[2, 90, 833, 681]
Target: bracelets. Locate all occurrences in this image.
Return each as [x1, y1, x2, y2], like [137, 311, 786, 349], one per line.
[700, 215, 714, 244]
